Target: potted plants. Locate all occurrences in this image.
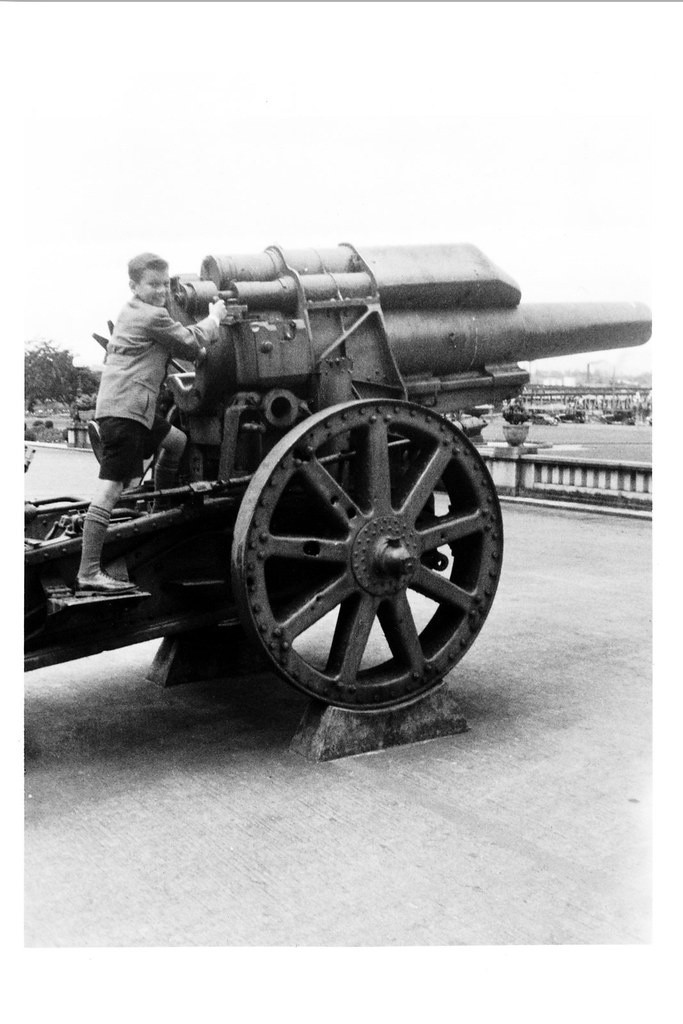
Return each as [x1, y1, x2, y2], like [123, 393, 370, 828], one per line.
[78, 394, 95, 422]
[501, 405, 530, 446]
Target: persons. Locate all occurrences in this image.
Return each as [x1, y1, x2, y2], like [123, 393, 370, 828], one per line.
[74, 253, 228, 599]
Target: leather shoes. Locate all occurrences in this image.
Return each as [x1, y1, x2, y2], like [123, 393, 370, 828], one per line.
[74, 572, 136, 598]
